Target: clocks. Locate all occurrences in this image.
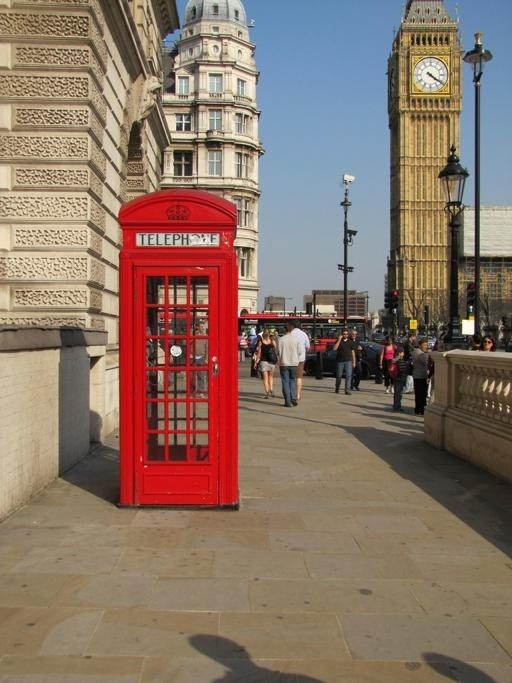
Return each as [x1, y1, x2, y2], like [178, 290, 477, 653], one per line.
[410, 55, 451, 94]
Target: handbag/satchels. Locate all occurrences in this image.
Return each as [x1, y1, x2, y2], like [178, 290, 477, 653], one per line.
[268, 339, 278, 364]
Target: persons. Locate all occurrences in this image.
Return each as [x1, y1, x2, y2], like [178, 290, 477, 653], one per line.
[350, 330, 366, 391]
[332, 329, 356, 395]
[379, 331, 445, 416]
[465, 331, 497, 352]
[238, 319, 311, 408]
[146, 318, 207, 399]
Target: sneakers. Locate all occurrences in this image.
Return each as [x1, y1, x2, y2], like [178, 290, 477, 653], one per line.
[391, 388, 394, 393]
[386, 389, 391, 393]
[392, 408, 404, 412]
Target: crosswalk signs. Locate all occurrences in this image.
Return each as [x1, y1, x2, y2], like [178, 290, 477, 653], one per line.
[467, 282, 477, 314]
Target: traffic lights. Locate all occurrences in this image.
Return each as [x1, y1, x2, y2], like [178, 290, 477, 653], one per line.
[383, 290, 399, 308]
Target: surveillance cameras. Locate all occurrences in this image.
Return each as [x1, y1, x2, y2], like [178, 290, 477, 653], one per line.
[347, 227, 357, 238]
[343, 174, 355, 182]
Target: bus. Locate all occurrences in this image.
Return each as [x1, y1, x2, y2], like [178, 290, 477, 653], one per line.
[238, 314, 367, 352]
[157, 312, 193, 344]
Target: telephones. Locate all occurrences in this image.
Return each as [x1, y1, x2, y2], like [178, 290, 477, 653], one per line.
[147, 327, 158, 376]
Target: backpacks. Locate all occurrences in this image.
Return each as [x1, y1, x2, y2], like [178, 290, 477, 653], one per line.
[387, 360, 403, 381]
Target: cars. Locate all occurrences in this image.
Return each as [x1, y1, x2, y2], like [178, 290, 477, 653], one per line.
[374, 331, 435, 349]
[304, 341, 387, 379]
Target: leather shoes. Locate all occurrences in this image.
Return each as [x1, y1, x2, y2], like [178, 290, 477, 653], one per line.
[264, 391, 297, 407]
[335, 384, 360, 395]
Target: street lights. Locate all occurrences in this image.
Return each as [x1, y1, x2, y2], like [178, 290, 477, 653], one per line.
[437, 142, 469, 342]
[462, 31, 493, 342]
